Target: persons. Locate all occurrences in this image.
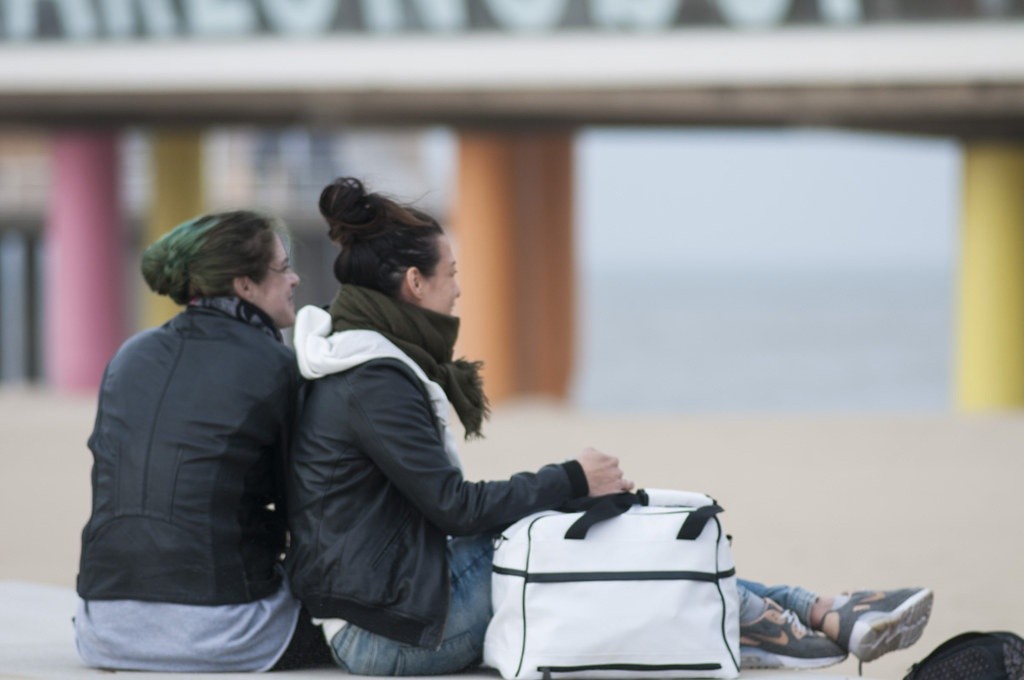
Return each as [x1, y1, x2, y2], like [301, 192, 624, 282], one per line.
[76, 211, 335, 669]
[286, 177, 933, 676]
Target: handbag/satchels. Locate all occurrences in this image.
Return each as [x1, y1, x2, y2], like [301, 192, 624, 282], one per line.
[480, 485, 742, 680]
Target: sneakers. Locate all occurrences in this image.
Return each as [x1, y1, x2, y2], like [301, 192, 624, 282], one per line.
[818, 585, 934, 661]
[740, 597, 847, 668]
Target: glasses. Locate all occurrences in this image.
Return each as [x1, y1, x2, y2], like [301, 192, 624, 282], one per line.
[263, 261, 292, 277]
